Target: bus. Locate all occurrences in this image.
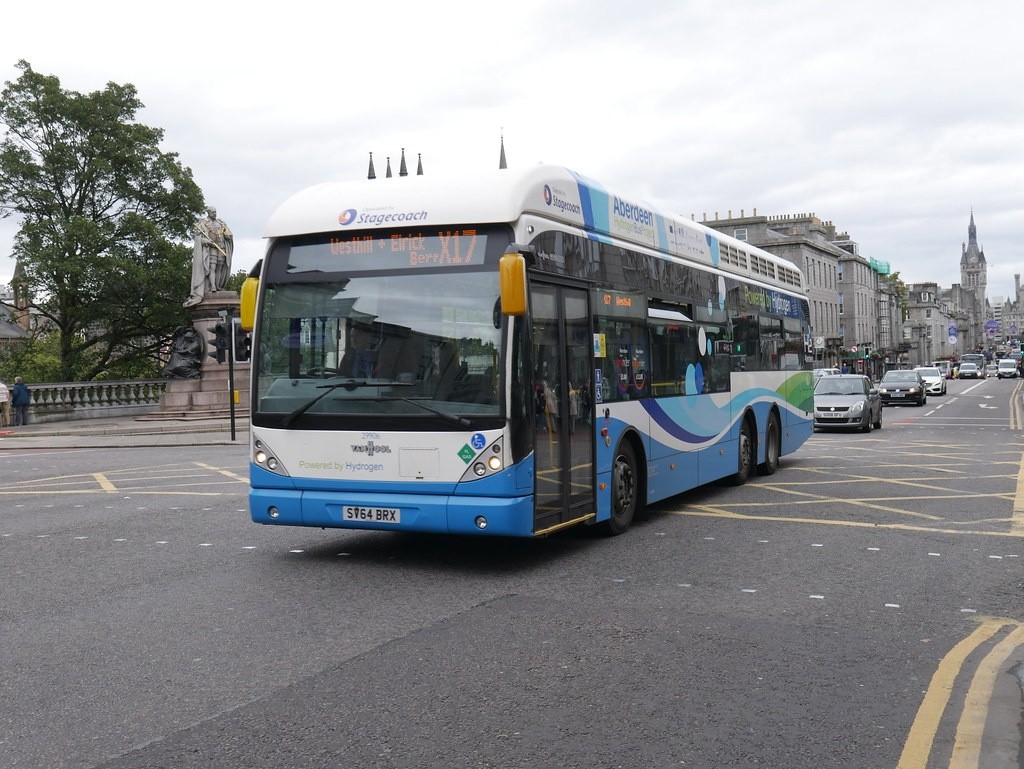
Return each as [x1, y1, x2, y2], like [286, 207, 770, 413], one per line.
[959, 353, 987, 379]
[240, 162, 817, 543]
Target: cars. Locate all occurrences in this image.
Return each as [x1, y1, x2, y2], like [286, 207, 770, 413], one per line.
[878, 369, 927, 406]
[813, 368, 844, 391]
[986, 365, 998, 376]
[813, 373, 883, 433]
[913, 367, 947, 396]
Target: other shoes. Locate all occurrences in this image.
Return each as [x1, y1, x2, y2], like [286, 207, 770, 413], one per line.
[3, 424, 10, 427]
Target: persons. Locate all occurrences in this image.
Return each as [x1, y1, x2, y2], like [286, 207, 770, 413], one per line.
[0, 381, 11, 427]
[539, 373, 594, 437]
[13, 376, 30, 426]
[164, 321, 200, 382]
[191, 207, 234, 292]
[338, 322, 392, 383]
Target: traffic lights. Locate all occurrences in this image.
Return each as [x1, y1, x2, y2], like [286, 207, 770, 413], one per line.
[216, 323, 230, 350]
[732, 325, 748, 357]
[863, 347, 870, 359]
[1020, 343, 1024, 355]
[234, 322, 252, 364]
[206, 326, 226, 364]
[1002, 340, 1006, 345]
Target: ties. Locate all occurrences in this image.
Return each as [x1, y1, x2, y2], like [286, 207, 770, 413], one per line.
[355, 351, 365, 378]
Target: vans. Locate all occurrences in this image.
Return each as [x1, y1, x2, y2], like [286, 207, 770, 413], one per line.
[997, 358, 1018, 379]
[959, 363, 980, 379]
[933, 361, 951, 379]
